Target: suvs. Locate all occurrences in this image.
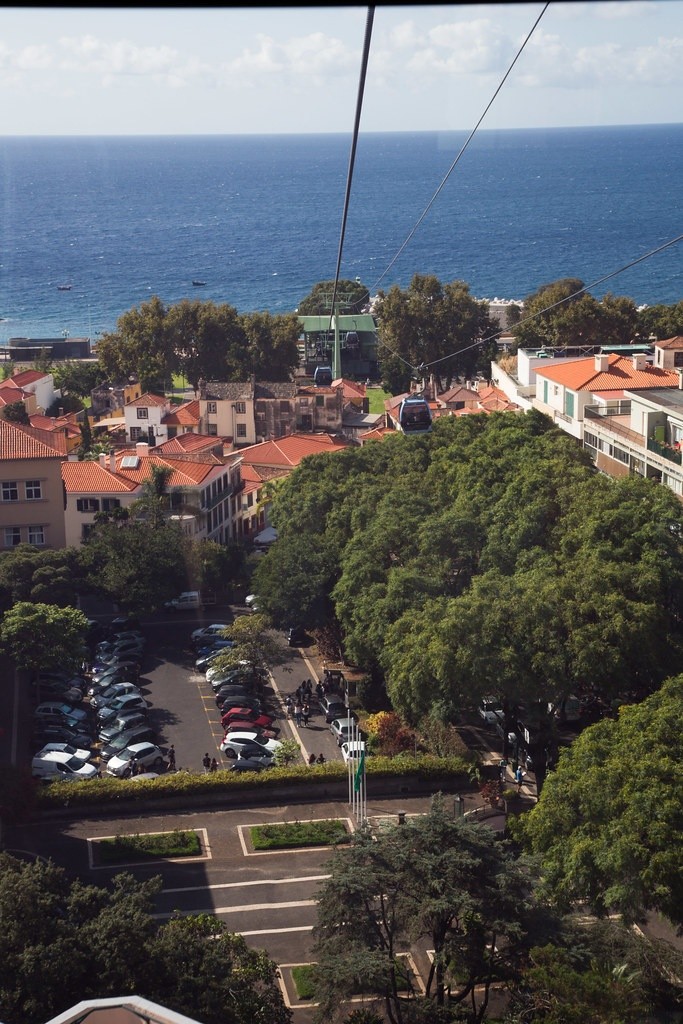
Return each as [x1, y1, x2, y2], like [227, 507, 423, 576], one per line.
[283, 628, 310, 648]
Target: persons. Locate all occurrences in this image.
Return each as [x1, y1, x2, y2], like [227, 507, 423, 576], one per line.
[202, 753, 217, 774]
[128, 756, 146, 776]
[516, 766, 526, 793]
[286, 678, 312, 727]
[316, 681, 329, 697]
[309, 754, 326, 764]
[499, 754, 507, 782]
[167, 745, 190, 774]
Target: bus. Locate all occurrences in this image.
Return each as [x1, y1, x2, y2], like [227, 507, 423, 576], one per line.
[314, 365, 332, 386]
[399, 396, 433, 436]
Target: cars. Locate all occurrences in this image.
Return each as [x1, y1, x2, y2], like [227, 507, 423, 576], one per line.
[341, 741, 366, 764]
[245, 594, 262, 607]
[190, 624, 286, 772]
[27, 616, 163, 782]
[330, 718, 360, 747]
[315, 693, 347, 723]
[251, 603, 268, 614]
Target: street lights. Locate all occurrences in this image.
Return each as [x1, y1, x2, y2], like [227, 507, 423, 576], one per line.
[453, 794, 465, 821]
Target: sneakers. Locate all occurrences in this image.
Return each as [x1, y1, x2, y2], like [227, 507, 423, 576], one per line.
[303, 724, 310, 728]
[296, 724, 301, 728]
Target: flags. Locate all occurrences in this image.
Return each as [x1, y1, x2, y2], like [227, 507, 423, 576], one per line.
[354, 752, 365, 791]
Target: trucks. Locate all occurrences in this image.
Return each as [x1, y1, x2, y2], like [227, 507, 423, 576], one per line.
[164, 589, 216, 612]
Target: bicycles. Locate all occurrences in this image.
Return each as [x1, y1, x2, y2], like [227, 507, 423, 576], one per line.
[300, 693, 310, 705]
